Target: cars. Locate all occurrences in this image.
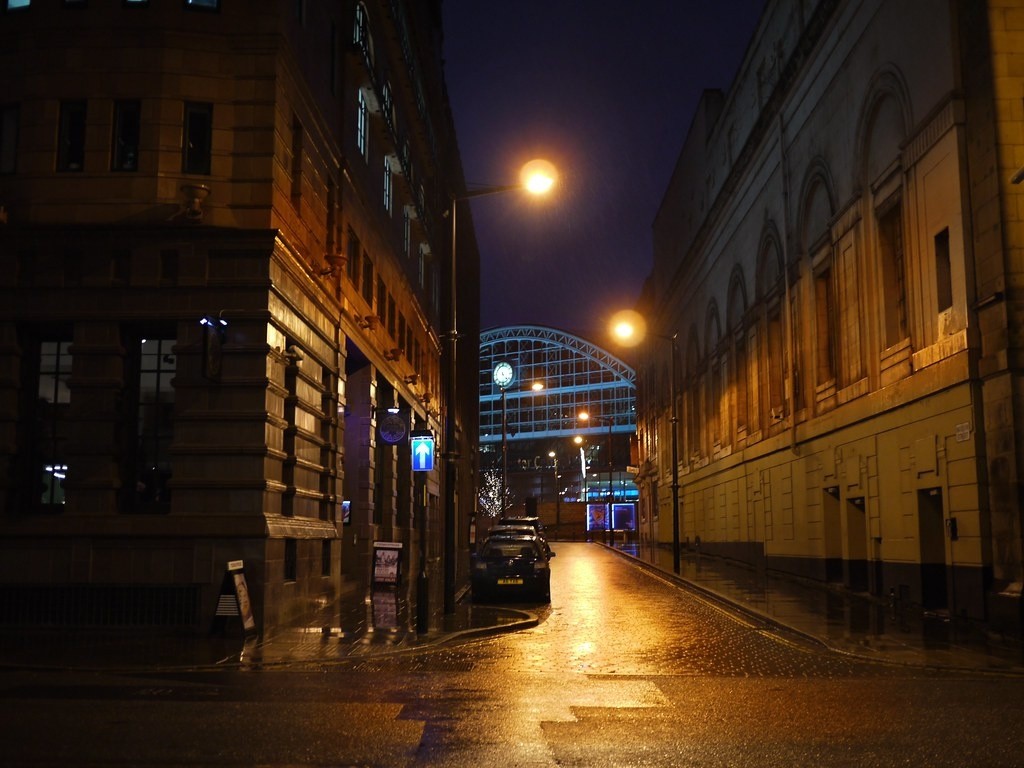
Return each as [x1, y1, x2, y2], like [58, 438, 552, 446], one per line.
[484, 514, 557, 564]
[469, 534, 556, 604]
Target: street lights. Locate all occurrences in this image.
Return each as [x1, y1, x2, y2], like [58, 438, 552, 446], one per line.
[607, 310, 680, 578]
[578, 412, 615, 546]
[441, 159, 562, 618]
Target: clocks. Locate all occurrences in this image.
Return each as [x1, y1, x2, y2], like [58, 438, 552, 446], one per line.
[493, 360, 516, 388]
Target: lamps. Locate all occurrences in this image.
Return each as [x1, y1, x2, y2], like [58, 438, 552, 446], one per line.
[420, 393, 433, 403]
[320, 255, 348, 277]
[432, 406, 447, 416]
[360, 316, 380, 330]
[179, 184, 211, 223]
[404, 374, 420, 385]
[384, 348, 403, 362]
